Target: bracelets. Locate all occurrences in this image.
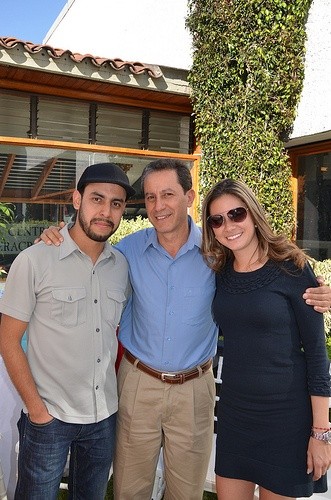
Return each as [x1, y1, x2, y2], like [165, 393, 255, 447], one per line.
[313, 426, 331, 431]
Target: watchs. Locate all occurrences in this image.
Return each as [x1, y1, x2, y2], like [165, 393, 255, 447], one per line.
[311, 431, 331, 445]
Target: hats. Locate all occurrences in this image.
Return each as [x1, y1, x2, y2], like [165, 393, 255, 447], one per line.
[77, 163, 138, 202]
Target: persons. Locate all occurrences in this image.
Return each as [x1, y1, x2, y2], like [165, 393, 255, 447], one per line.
[34, 159, 331, 500]
[200, 179, 331, 500]
[0, 162, 134, 500]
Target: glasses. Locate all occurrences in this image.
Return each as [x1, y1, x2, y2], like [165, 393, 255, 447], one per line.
[206, 207, 249, 228]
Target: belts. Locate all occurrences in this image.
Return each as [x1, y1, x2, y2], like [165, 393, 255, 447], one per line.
[123, 348, 213, 384]
